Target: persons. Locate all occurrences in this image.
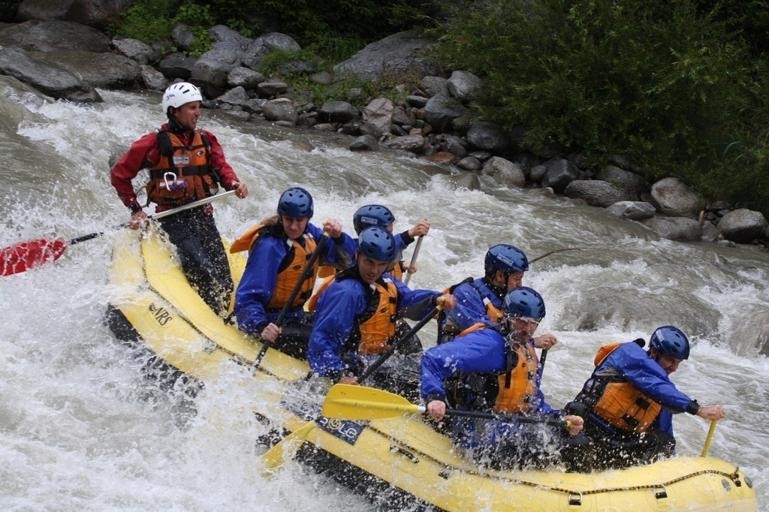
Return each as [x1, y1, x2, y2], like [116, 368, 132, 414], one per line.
[350, 204, 431, 285]
[308, 226, 456, 404]
[562, 325, 726, 475]
[228, 188, 342, 359]
[421, 287, 583, 458]
[436, 243, 557, 352]
[110, 81, 248, 324]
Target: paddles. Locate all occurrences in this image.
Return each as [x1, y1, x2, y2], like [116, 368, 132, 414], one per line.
[322, 383, 573, 429]
[1, 188, 240, 278]
[259, 303, 446, 477]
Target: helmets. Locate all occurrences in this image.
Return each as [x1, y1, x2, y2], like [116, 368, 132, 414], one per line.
[502, 286, 546, 317]
[483, 244, 529, 276]
[352, 204, 395, 237]
[358, 225, 395, 260]
[160, 82, 203, 114]
[648, 325, 689, 360]
[276, 187, 315, 219]
